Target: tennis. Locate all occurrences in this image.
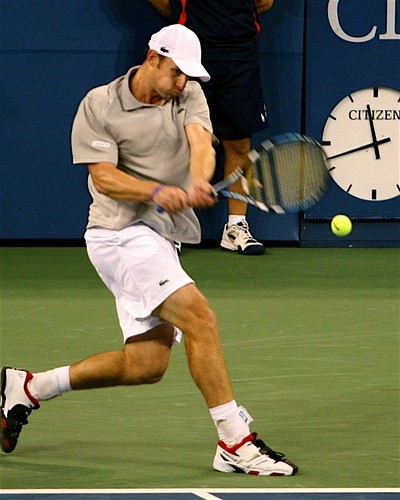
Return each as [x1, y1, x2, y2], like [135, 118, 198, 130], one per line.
[331, 215, 352, 238]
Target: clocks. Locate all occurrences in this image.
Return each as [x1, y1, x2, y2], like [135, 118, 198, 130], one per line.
[320, 85, 400, 202]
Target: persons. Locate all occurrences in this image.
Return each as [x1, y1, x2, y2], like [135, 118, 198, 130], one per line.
[153, 0, 272, 256]
[0, 23, 297, 477]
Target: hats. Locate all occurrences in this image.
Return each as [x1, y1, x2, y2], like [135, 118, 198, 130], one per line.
[149, 24, 211, 83]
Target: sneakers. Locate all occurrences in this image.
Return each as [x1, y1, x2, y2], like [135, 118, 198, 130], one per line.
[212, 433, 299, 477]
[0, 366, 39, 452]
[221, 221, 266, 254]
